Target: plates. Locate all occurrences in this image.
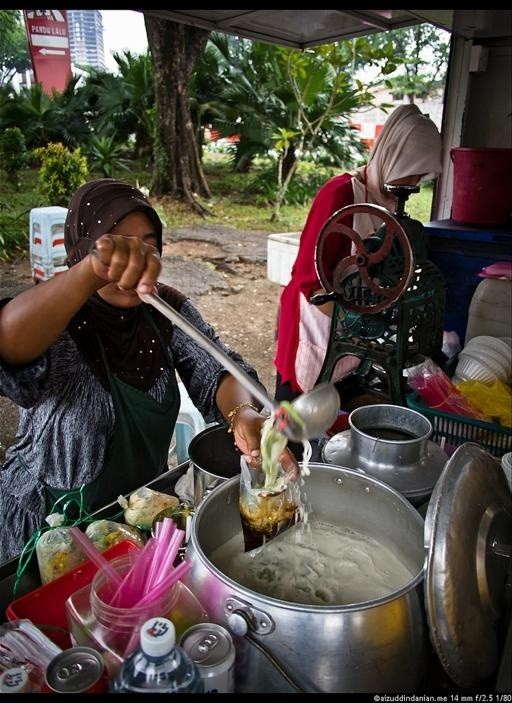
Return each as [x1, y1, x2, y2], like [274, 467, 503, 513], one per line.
[8, 540, 144, 652]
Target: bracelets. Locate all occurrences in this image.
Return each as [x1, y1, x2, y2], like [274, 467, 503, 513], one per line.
[226, 401, 259, 434]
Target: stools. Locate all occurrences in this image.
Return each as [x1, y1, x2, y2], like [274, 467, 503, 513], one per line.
[29, 205, 70, 283]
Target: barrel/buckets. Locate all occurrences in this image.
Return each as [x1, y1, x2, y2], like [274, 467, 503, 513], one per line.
[462, 263, 512, 343]
[450, 148, 512, 225]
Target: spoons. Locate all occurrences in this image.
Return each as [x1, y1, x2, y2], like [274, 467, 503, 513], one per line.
[92, 247, 340, 440]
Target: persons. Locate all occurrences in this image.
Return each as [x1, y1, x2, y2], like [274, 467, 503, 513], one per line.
[0, 177, 300, 568]
[274, 103, 445, 406]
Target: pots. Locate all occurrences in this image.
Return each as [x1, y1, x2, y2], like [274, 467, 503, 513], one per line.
[186, 442, 428, 694]
[188, 426, 311, 504]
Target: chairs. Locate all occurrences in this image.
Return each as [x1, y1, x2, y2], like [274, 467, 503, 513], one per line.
[168, 384, 218, 471]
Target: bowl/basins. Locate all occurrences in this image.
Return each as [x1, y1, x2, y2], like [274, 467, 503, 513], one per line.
[452, 335, 512, 387]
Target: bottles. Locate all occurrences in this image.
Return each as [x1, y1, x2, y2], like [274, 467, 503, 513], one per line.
[43, 646, 107, 693]
[0, 668, 39, 692]
[178, 624, 235, 693]
[114, 617, 195, 693]
